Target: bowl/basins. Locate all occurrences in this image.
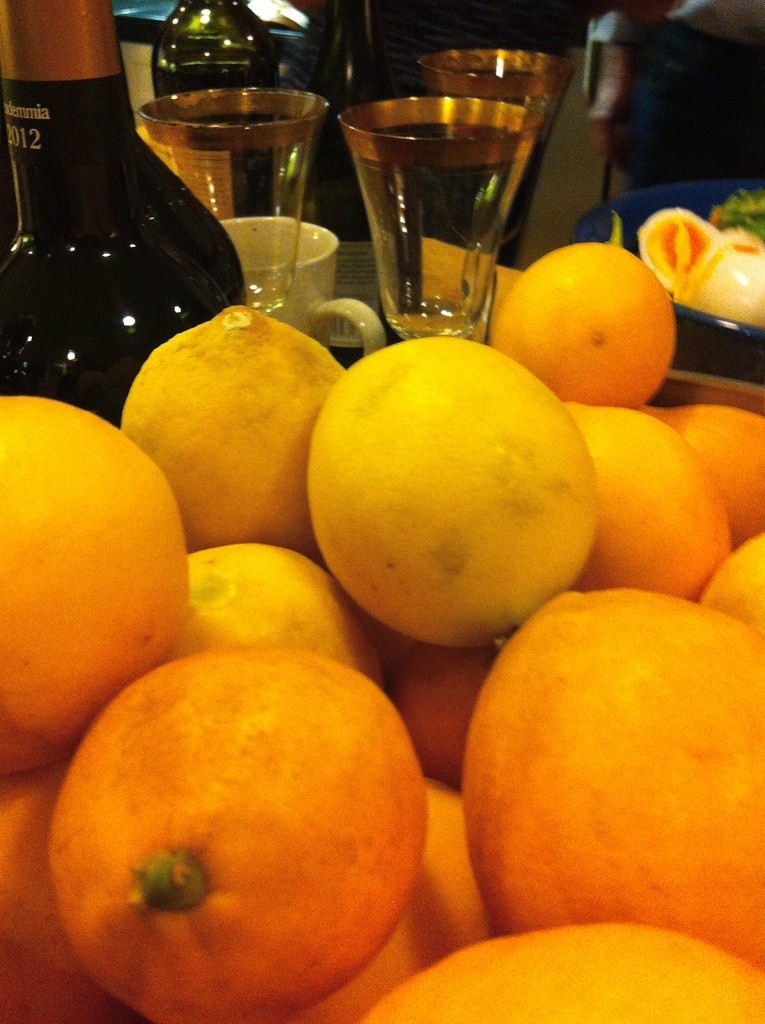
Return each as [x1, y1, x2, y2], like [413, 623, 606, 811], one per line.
[2, 370, 765, 613]
[570, 179, 764, 387]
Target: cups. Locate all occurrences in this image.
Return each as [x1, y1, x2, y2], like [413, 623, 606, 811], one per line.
[219, 216, 387, 355]
[337, 95, 528, 342]
[417, 48, 575, 248]
[137, 87, 329, 317]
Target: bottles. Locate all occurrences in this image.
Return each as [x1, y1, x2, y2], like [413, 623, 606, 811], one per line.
[0, 0, 245, 431]
[150, 1, 278, 217]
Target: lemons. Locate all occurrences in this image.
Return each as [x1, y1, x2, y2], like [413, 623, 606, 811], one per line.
[0, 238, 765, 1024]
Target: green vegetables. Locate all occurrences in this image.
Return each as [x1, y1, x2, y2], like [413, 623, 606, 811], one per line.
[711, 185, 765, 243]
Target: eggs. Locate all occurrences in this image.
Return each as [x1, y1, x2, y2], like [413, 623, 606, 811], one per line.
[684, 228, 765, 327]
[636, 206, 721, 294]
